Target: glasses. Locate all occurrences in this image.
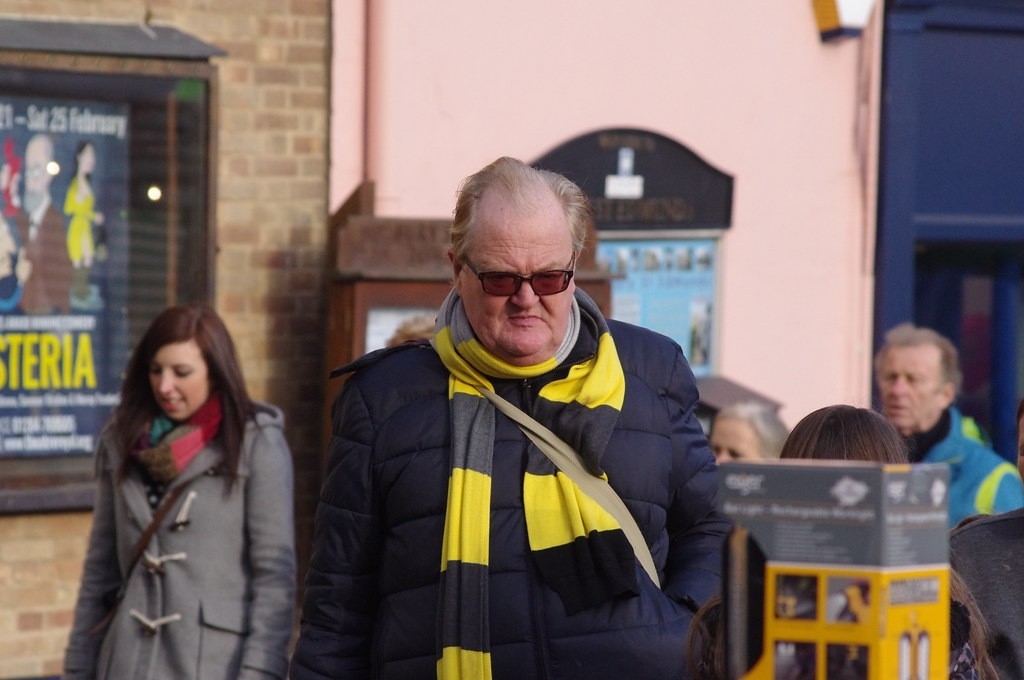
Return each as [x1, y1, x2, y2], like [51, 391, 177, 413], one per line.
[462, 252, 576, 296]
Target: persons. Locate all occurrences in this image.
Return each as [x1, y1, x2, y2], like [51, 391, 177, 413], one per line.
[686, 405, 911, 680]
[62, 303, 298, 680]
[707, 402, 788, 461]
[287, 156, 733, 679]
[875, 322, 1024, 527]
[949, 399, 1024, 679]
